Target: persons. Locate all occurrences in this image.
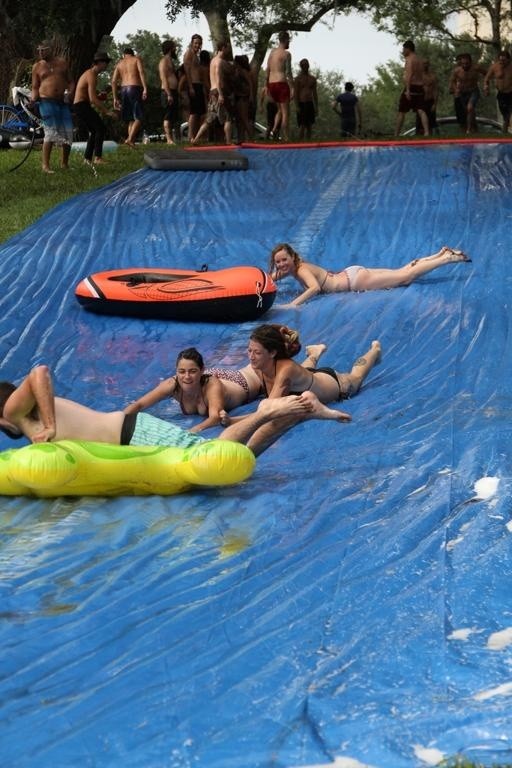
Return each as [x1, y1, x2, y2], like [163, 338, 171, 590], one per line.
[121, 346, 264, 435]
[393, 41, 438, 137]
[332, 82, 361, 139]
[11, 26, 318, 175]
[218, 322, 382, 431]
[0, 365, 352, 459]
[447, 51, 512, 137]
[267, 242, 474, 311]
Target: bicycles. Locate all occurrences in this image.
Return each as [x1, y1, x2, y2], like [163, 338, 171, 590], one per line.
[0, 84, 39, 175]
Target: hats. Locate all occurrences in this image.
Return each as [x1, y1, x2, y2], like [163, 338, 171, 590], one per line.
[93, 52, 112, 62]
[277, 32, 289, 38]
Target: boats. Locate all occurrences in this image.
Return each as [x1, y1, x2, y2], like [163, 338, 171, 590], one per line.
[1, 441, 262, 501]
[141, 145, 253, 173]
[73, 263, 278, 326]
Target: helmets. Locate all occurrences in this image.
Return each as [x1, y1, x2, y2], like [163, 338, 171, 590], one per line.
[37, 40, 53, 59]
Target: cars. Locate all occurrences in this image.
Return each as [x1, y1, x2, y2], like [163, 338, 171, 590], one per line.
[144, 119, 281, 142]
[400, 116, 512, 142]
